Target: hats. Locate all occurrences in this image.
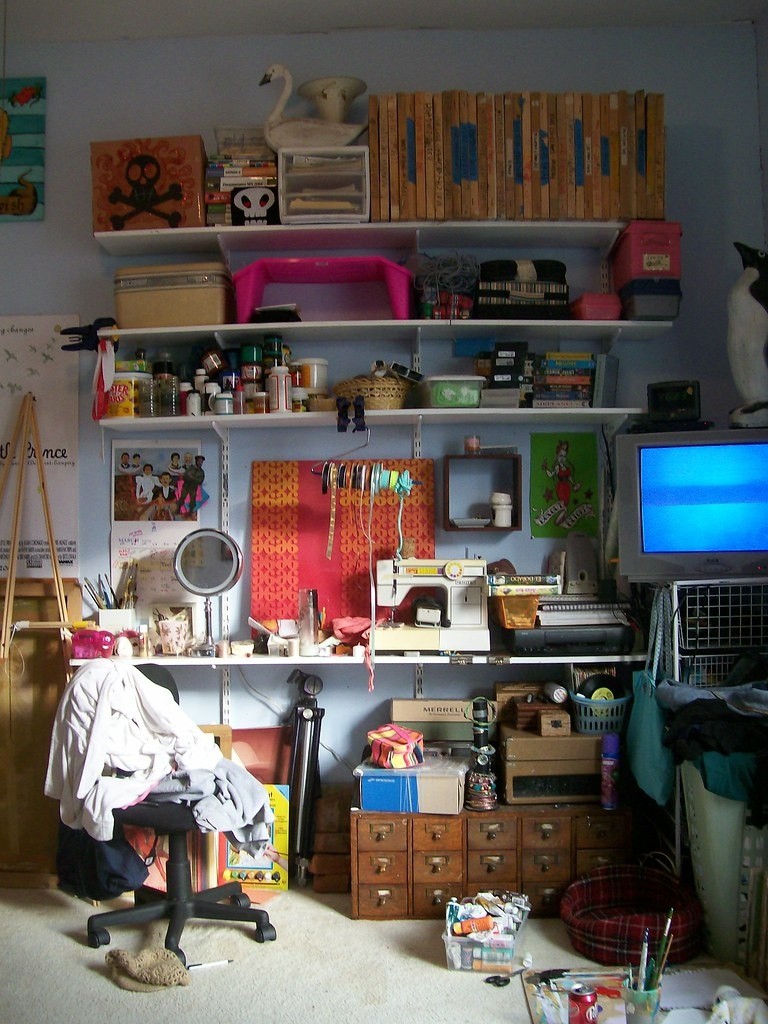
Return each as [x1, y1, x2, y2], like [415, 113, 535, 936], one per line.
[106, 949, 187, 991]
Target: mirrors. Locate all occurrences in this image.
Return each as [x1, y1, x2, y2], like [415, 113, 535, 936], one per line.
[173, 529, 244, 657]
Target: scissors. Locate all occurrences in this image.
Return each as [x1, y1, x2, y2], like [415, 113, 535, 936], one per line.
[486, 967, 528, 987]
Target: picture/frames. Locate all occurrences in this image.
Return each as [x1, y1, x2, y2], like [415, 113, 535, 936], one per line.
[148, 602, 195, 656]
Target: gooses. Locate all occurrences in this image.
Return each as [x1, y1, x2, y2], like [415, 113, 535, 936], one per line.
[258, 64, 369, 154]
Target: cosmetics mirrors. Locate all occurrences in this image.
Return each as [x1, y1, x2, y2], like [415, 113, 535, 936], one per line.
[173, 528, 243, 656]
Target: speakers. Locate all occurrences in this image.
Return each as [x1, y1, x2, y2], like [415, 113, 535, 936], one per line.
[564, 539, 600, 593]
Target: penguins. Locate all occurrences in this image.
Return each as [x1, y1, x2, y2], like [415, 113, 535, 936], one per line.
[726, 241, 768, 402]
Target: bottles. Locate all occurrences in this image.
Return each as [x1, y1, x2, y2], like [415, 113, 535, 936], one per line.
[523, 953, 533, 967]
[452, 916, 514, 973]
[180, 336, 327, 415]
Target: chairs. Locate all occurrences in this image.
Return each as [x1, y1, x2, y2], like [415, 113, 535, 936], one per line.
[45, 658, 276, 970]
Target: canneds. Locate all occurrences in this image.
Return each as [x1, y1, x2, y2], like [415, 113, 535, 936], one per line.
[422, 286, 474, 319]
[202, 335, 282, 413]
[567, 983, 598, 1024]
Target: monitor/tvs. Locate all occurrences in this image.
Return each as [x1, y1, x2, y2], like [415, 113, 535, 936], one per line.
[614, 428, 768, 582]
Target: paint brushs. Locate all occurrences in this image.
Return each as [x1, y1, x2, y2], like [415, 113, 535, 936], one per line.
[118, 558, 139, 609]
[629, 907, 674, 991]
[83, 574, 121, 609]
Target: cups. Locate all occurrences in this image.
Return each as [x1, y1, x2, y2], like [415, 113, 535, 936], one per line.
[159, 375, 180, 416]
[136, 378, 159, 417]
[464, 434, 480, 456]
[623, 978, 662, 1024]
[299, 589, 319, 656]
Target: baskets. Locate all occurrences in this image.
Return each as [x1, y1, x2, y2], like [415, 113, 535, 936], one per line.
[330, 377, 412, 410]
[569, 689, 631, 734]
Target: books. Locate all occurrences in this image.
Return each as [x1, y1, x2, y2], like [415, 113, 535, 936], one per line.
[487, 574, 629, 626]
[205, 156, 277, 223]
[287, 155, 362, 211]
[475, 351, 618, 408]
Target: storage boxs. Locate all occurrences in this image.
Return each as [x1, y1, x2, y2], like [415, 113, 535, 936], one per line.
[613, 221, 683, 319]
[488, 596, 539, 629]
[90, 135, 209, 231]
[353, 742, 473, 815]
[441, 903, 532, 973]
[116, 360, 154, 373]
[500, 721, 603, 805]
[572, 294, 622, 320]
[389, 698, 499, 741]
[494, 681, 571, 737]
[112, 263, 233, 329]
[215, 125, 275, 160]
[411, 375, 521, 408]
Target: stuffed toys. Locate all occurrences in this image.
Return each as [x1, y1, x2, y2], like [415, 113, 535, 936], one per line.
[727, 242, 768, 428]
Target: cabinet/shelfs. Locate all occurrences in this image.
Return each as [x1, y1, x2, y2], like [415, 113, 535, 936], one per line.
[96, 223, 672, 431]
[641, 577, 768, 877]
[350, 787, 634, 920]
[277, 145, 371, 222]
[443, 454, 522, 531]
